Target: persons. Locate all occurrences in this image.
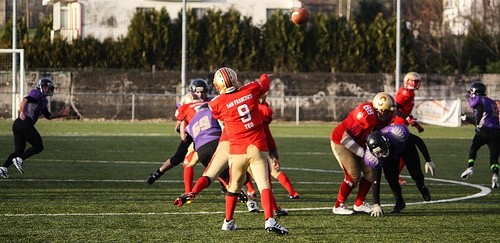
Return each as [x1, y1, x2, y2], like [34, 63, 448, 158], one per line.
[148, 79, 300, 218]
[0, 78, 71, 179]
[208, 68, 290, 235]
[330, 92, 397, 214]
[365, 124, 434, 217]
[389, 72, 424, 185]
[460, 83, 500, 188]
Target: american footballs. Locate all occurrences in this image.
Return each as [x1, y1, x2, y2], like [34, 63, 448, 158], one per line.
[292, 8, 311, 24]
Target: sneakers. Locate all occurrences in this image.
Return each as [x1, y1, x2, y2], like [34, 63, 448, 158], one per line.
[0, 167, 10, 179]
[461, 167, 474, 180]
[332, 202, 354, 214]
[273, 209, 288, 216]
[353, 202, 373, 214]
[221, 218, 237, 231]
[173, 192, 195, 206]
[12, 157, 24, 174]
[491, 173, 500, 189]
[265, 217, 289, 235]
[221, 185, 260, 213]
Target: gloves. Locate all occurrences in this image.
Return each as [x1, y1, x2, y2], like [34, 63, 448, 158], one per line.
[370, 203, 384, 217]
[414, 122, 424, 133]
[425, 161, 436, 176]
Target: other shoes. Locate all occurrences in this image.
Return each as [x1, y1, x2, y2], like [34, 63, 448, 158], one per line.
[286, 192, 300, 199]
[146, 173, 158, 185]
[393, 201, 406, 213]
[417, 184, 431, 201]
[399, 177, 407, 185]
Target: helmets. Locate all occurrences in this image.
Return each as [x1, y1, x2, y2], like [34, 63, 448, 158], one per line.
[213, 67, 237, 94]
[468, 81, 488, 96]
[372, 92, 396, 121]
[367, 130, 390, 158]
[404, 71, 422, 90]
[189, 78, 208, 100]
[36, 78, 55, 96]
